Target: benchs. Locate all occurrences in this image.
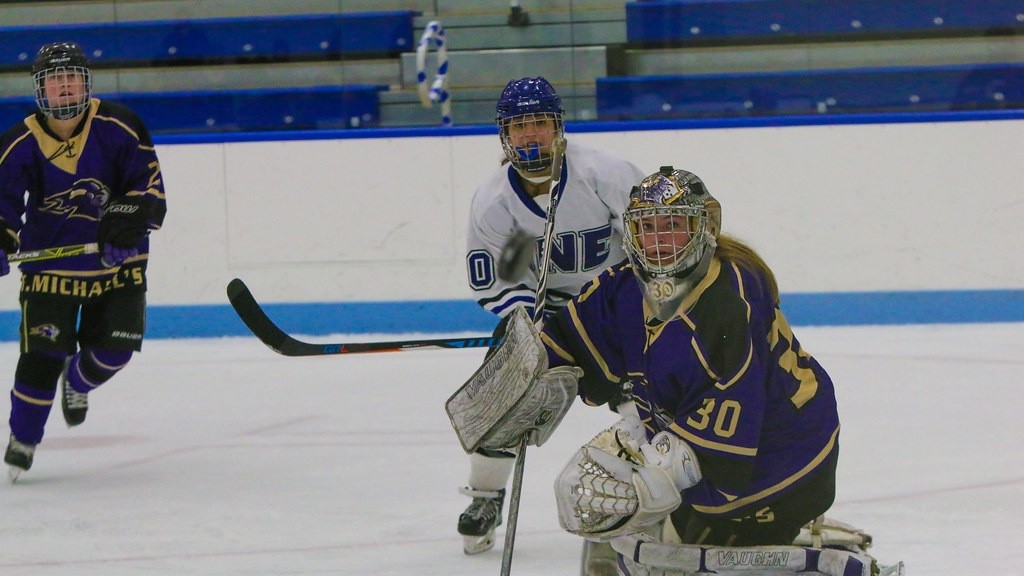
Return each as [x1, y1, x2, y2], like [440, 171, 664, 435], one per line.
[595, 0, 1024, 123]
[0, 0, 428, 135]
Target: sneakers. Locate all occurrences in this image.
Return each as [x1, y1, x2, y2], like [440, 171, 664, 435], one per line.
[4, 432, 36, 483]
[457, 486, 505, 553]
[61, 355, 89, 430]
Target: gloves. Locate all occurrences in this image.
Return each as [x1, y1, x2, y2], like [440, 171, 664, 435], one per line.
[0, 247, 10, 276]
[97, 220, 147, 266]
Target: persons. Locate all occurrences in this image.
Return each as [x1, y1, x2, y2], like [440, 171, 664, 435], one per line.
[0, 43, 168, 483]
[532, 164, 840, 575]
[457, 78, 649, 555]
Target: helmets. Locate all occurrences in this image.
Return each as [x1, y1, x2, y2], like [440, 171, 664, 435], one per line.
[496, 76, 566, 183]
[31, 40, 94, 121]
[621, 166, 722, 321]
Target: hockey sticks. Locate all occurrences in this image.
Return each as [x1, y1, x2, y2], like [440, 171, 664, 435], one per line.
[225, 276, 506, 360]
[6, 241, 101, 263]
[497, 136, 569, 576]
